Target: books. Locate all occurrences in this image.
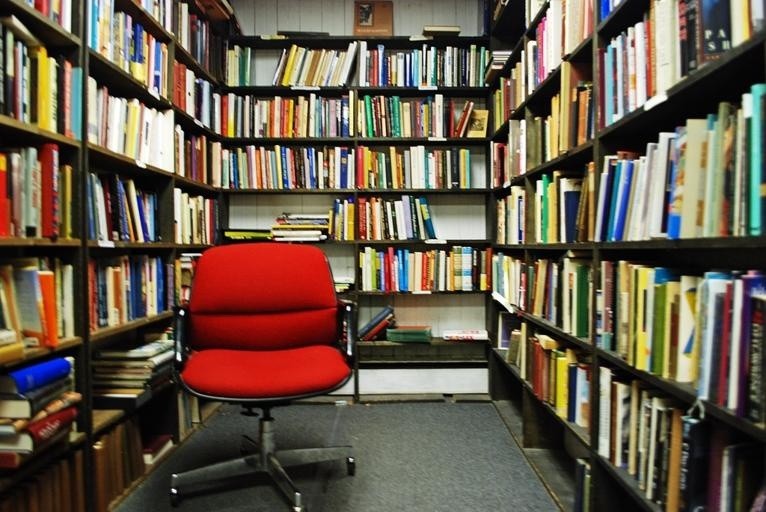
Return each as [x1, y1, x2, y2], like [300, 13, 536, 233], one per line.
[1, 0, 225, 188]
[593, 1, 765, 509]
[0, 143, 222, 511]
[229, 89, 489, 139]
[332, 245, 493, 344]
[488, 161, 592, 431]
[226, 43, 491, 88]
[481, 0, 595, 188]
[226, 196, 437, 242]
[224, 144, 472, 190]
